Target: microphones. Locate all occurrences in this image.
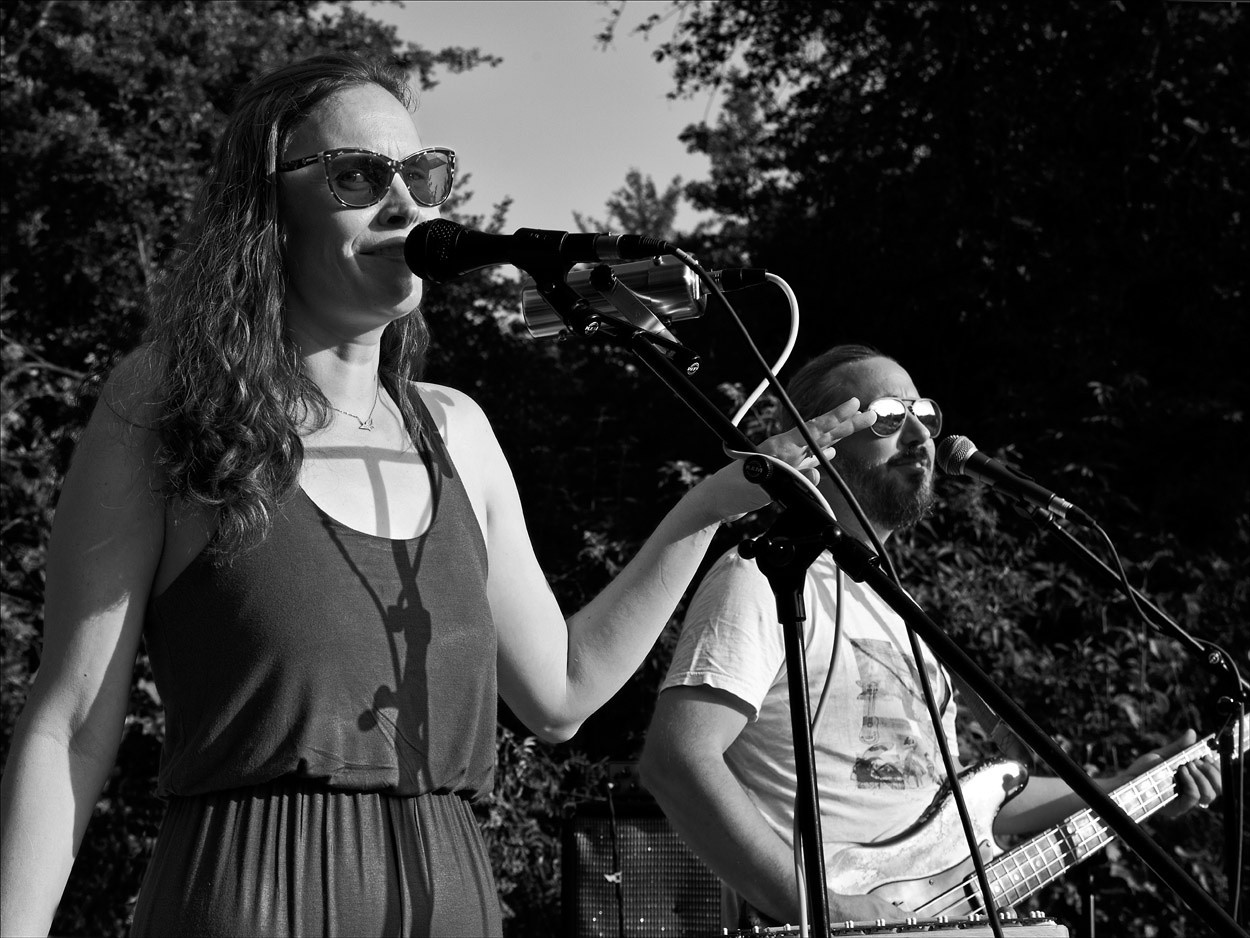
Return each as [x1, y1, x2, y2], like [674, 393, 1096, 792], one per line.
[936, 435, 1096, 529]
[521, 253, 768, 339]
[403, 218, 670, 283]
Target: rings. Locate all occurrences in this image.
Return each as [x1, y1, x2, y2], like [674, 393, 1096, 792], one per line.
[808, 447, 822, 461]
[1197, 800, 1209, 812]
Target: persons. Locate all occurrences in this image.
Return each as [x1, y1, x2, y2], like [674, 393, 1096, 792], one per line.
[0, 54, 877, 937]
[636, 344, 1227, 938]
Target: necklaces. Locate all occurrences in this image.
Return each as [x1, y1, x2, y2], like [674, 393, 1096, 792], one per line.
[301, 370, 395, 432]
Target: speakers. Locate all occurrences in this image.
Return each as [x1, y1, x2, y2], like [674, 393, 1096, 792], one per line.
[561, 801, 750, 938]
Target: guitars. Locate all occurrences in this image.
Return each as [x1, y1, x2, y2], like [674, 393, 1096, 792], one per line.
[865, 714, 1250, 926]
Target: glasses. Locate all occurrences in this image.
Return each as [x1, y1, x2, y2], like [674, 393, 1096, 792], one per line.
[868, 398, 943, 438]
[278, 147, 455, 208]
